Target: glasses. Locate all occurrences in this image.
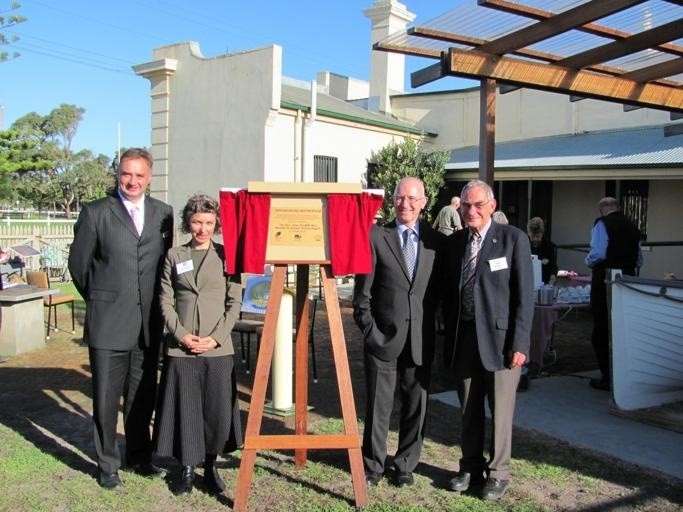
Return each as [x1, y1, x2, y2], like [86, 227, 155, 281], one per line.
[390, 192, 427, 207]
[460, 199, 492, 210]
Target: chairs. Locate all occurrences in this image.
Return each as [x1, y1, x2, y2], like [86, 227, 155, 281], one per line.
[257, 299, 318, 384]
[27, 271, 76, 340]
[232, 288, 264, 374]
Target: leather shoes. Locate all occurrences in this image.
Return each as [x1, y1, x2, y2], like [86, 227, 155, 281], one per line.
[589, 377, 611, 393]
[393, 469, 412, 489]
[479, 475, 512, 502]
[95, 463, 123, 489]
[447, 467, 481, 492]
[132, 461, 162, 478]
[362, 471, 383, 487]
[204, 460, 226, 493]
[180, 462, 197, 493]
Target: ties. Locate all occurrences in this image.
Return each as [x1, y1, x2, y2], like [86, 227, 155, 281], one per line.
[130, 206, 145, 235]
[401, 229, 419, 285]
[459, 233, 478, 316]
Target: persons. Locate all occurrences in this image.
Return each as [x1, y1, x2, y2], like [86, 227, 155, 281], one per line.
[526, 218, 558, 287]
[0, 247, 10, 366]
[492, 212, 507, 225]
[432, 198, 464, 235]
[443, 180, 534, 501]
[69, 150, 172, 491]
[150, 195, 246, 496]
[354, 182, 447, 487]
[585, 202, 640, 391]
